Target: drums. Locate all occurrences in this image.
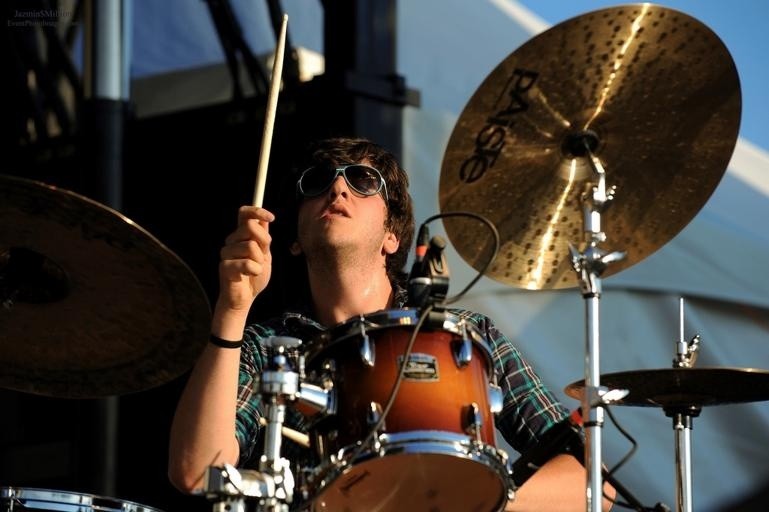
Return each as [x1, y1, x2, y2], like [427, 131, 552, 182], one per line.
[0, 486, 160, 512]
[287, 309, 514, 511]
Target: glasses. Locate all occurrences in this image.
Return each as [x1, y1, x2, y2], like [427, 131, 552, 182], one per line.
[296, 164, 389, 204]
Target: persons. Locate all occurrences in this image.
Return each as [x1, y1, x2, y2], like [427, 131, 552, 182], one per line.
[165, 134, 620, 512]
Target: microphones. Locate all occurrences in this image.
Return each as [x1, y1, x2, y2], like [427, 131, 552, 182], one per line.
[513, 406, 584, 486]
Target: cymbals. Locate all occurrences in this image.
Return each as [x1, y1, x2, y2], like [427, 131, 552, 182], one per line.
[1, 178, 212, 398]
[564, 367, 769, 406]
[438, 4, 742, 292]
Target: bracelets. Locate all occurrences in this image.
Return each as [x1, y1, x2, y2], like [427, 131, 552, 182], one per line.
[200, 329, 244, 351]
